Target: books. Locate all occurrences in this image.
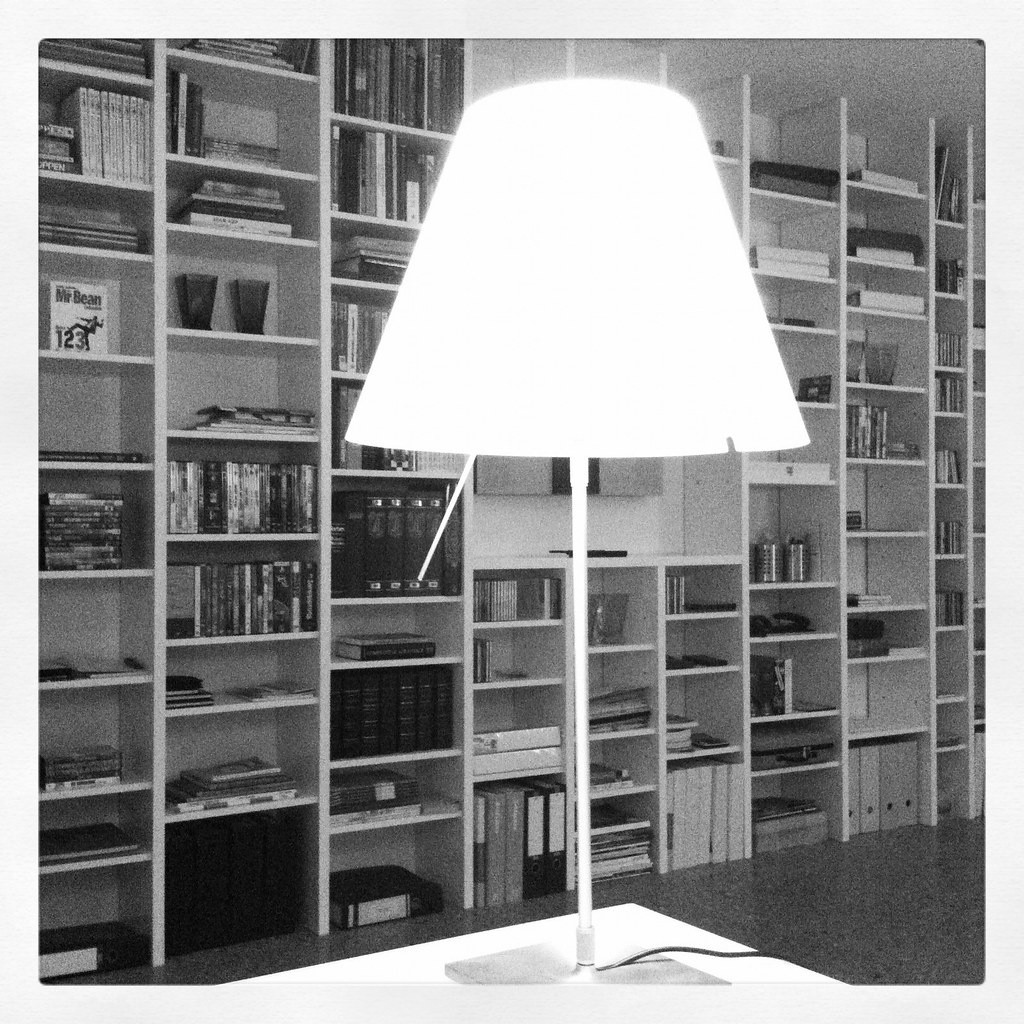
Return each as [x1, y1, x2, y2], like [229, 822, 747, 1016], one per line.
[38, 38, 465, 283]
[473, 575, 743, 907]
[329, 301, 388, 374]
[39, 387, 464, 980]
[746, 141, 985, 856]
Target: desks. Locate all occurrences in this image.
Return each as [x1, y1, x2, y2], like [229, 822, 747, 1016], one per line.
[202, 901, 854, 986]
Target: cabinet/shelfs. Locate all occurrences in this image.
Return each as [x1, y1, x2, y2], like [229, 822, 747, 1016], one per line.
[39, 38, 986, 984]
[468, 549, 754, 911]
